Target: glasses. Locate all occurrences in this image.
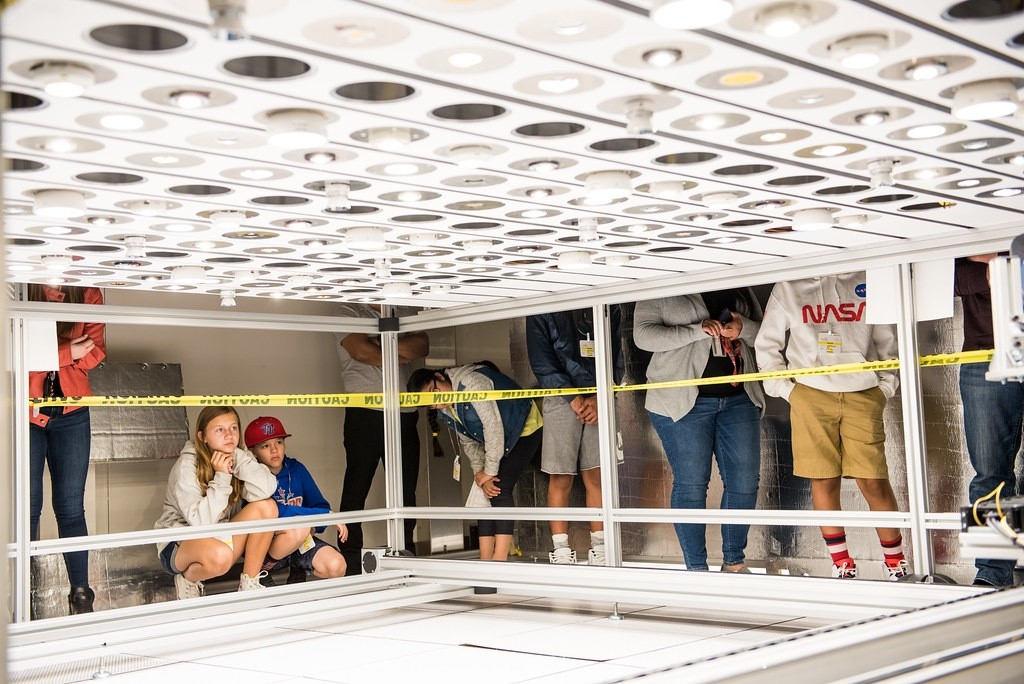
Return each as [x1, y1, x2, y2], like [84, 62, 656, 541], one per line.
[428, 377, 441, 410]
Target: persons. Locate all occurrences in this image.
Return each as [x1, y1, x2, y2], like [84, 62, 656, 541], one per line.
[755, 271, 909, 581]
[327, 304, 430, 577]
[954, 250, 1024, 586]
[153, 406, 279, 601]
[26, 283, 106, 615]
[633, 287, 766, 573]
[526, 304, 626, 566]
[244, 416, 348, 589]
[407, 360, 543, 561]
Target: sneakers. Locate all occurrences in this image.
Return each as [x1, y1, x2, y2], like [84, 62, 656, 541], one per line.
[587, 548, 606, 565]
[174, 573, 203, 600]
[238, 570, 268, 591]
[549, 547, 577, 565]
[884, 560, 909, 581]
[831, 558, 857, 579]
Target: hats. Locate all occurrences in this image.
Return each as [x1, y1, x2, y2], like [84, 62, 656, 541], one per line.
[244, 415, 292, 449]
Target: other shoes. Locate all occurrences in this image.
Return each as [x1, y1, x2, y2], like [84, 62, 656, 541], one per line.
[259, 569, 277, 587]
[720, 563, 752, 574]
[972, 579, 993, 586]
[286, 561, 306, 585]
[69, 586, 94, 614]
